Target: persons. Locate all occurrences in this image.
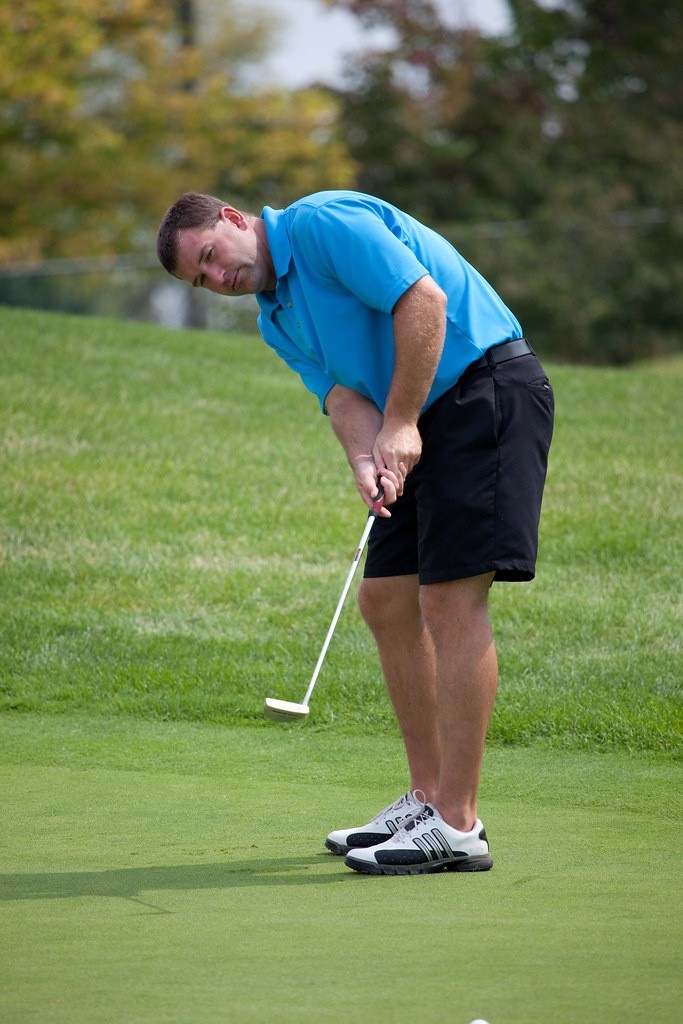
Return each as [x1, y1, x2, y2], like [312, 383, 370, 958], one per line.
[157, 191, 555, 875]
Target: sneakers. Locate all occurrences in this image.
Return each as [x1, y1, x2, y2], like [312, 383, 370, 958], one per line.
[324, 790, 426, 855]
[344, 802, 493, 875]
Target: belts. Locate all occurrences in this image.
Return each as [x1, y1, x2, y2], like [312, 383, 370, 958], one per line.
[470, 338, 531, 369]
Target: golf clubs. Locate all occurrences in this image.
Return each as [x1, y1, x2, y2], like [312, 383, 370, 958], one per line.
[263, 477, 385, 723]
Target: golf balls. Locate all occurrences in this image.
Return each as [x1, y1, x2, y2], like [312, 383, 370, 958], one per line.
[470, 1019, 489, 1024]
